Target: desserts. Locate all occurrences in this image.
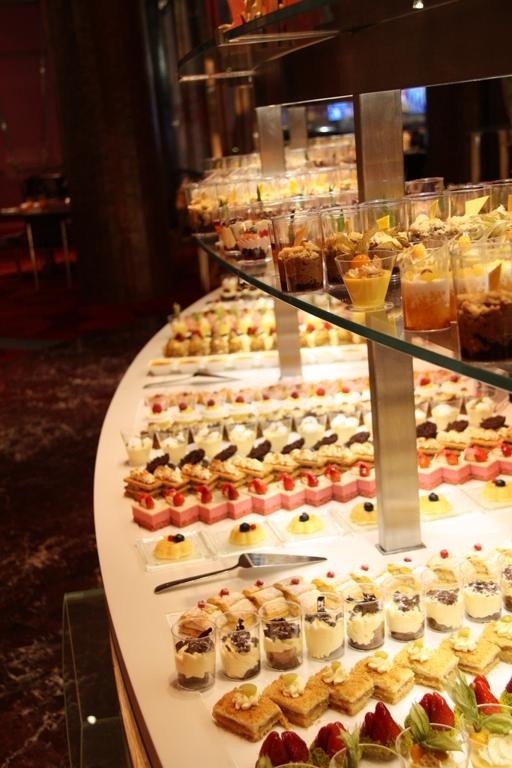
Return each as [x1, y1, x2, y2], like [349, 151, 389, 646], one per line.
[181, 131, 512, 362]
[125, 276, 512, 768]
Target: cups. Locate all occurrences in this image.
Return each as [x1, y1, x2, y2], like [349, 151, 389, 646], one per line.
[460, 703, 512, 768]
[191, 422, 224, 458]
[468, 559, 502, 623]
[399, 240, 452, 333]
[344, 586, 386, 651]
[120, 428, 151, 465]
[294, 415, 327, 445]
[329, 410, 361, 442]
[225, 423, 258, 456]
[188, 203, 220, 231]
[447, 186, 488, 218]
[428, 566, 466, 631]
[259, 416, 292, 453]
[430, 398, 462, 426]
[489, 180, 511, 210]
[362, 401, 373, 442]
[272, 214, 327, 293]
[464, 395, 495, 421]
[390, 575, 426, 643]
[335, 249, 400, 308]
[415, 394, 428, 425]
[322, 204, 369, 288]
[366, 199, 408, 232]
[275, 762, 319, 768]
[173, 619, 217, 692]
[407, 177, 444, 192]
[217, 608, 262, 680]
[260, 599, 302, 671]
[155, 428, 189, 463]
[228, 219, 268, 259]
[405, 192, 445, 223]
[395, 723, 469, 768]
[330, 743, 407, 768]
[304, 593, 346, 661]
[451, 240, 512, 361]
[217, 190, 359, 240]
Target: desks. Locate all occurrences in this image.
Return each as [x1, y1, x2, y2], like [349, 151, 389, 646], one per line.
[0, 206, 76, 297]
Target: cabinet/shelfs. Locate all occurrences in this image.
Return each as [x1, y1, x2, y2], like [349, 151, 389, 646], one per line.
[91, 0, 512, 768]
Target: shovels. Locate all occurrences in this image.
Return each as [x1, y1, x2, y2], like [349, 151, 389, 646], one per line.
[154, 553, 327, 593]
[143, 369, 240, 389]
